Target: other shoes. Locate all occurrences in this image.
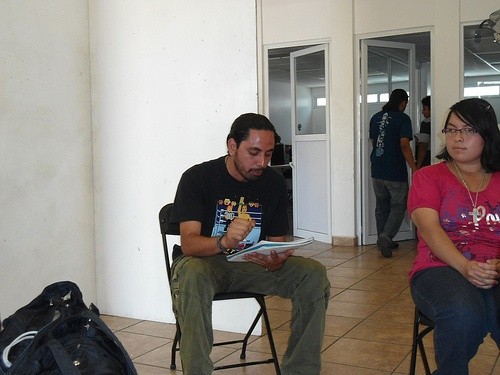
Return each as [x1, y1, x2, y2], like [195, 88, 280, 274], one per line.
[380, 245, 399, 258]
[377, 241, 399, 249]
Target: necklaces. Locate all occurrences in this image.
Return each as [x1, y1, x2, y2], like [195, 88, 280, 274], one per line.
[453, 160, 485, 224]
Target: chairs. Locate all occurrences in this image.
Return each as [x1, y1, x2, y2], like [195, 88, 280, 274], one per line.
[409, 297, 434, 375]
[158, 203, 281, 375]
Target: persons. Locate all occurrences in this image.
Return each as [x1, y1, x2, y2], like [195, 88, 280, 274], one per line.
[417, 96, 431, 169]
[170, 113, 331, 375]
[408, 98, 500, 375]
[369, 89, 415, 257]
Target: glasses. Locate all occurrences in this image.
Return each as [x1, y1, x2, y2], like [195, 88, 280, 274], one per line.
[441, 126, 477, 135]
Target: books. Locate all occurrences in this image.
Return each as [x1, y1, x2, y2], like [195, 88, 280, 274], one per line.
[226, 236, 314, 263]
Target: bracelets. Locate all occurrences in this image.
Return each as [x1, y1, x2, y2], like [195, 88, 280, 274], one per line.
[217, 234, 226, 251]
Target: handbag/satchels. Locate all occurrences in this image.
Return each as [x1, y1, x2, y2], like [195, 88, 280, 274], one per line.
[0, 280, 105, 375]
[4, 310, 142, 375]
